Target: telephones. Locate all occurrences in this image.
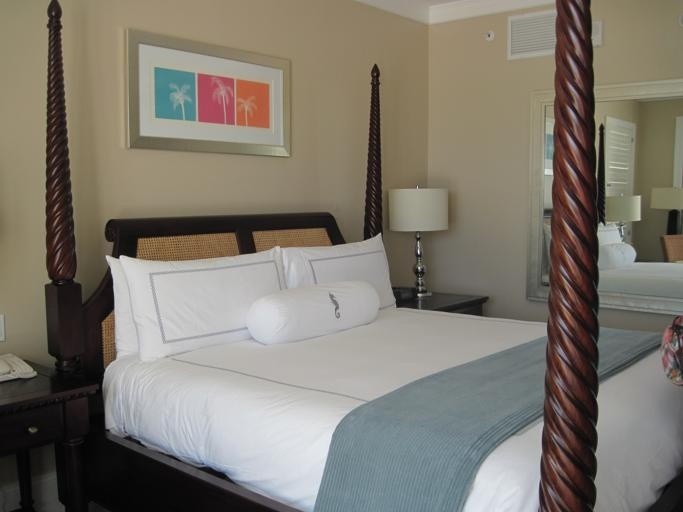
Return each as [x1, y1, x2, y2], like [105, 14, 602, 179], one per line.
[0, 353, 38, 383]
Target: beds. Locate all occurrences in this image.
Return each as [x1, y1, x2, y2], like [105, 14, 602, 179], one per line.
[540, 123, 682, 300]
[45, 0, 682, 512]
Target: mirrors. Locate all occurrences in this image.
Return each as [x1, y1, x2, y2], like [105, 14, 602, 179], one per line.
[525, 78, 682, 317]
[545, 115, 555, 176]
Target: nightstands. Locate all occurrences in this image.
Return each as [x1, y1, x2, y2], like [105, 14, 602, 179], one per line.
[0, 357, 100, 512]
[392, 287, 489, 317]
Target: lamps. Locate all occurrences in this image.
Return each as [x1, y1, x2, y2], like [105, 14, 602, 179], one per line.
[387, 184, 450, 296]
[649, 185, 682, 234]
[604, 191, 642, 239]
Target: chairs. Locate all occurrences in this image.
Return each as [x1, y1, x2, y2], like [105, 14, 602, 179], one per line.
[660, 234, 683, 264]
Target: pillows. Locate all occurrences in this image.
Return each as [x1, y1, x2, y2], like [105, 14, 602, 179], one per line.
[280, 233, 398, 310]
[247, 280, 382, 344]
[118, 246, 288, 363]
[544, 230, 552, 272]
[595, 243, 636, 270]
[595, 223, 623, 245]
[105, 255, 140, 361]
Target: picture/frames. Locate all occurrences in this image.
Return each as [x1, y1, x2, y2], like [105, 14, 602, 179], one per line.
[124, 25, 294, 158]
